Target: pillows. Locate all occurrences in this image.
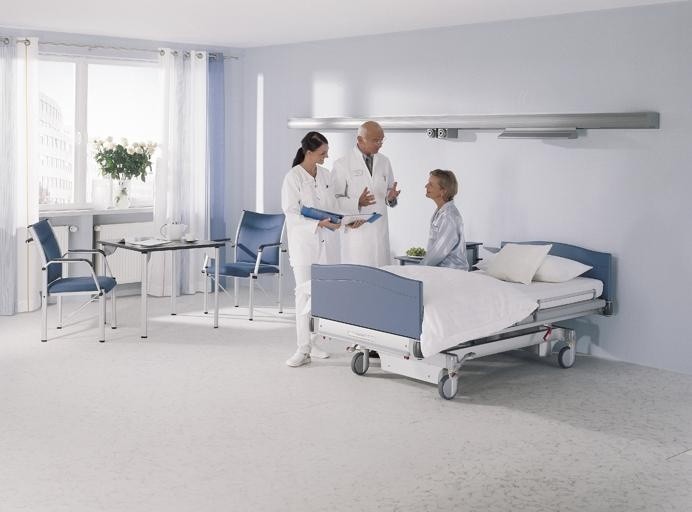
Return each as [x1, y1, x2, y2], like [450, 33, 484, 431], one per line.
[472, 243, 552, 286]
[532, 255, 593, 283]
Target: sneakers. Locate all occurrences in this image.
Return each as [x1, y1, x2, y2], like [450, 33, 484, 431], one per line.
[288, 354, 311, 366]
[312, 350, 329, 359]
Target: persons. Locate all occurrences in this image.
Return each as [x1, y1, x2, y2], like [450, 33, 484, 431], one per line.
[418, 168, 471, 272]
[329, 120, 402, 268]
[280, 130, 368, 368]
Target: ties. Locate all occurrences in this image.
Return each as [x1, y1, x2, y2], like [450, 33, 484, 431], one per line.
[367, 156, 373, 175]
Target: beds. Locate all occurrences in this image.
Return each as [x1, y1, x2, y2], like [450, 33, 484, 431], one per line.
[310, 241, 614, 400]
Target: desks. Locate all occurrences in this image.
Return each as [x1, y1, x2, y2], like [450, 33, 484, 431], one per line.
[96, 238, 225, 338]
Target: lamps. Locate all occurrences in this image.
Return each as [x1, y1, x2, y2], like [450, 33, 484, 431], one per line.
[498, 128, 577, 139]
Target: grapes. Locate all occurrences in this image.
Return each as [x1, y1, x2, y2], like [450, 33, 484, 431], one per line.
[407, 248, 426, 257]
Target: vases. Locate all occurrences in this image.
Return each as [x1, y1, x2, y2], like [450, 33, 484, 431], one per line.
[114, 186, 130, 209]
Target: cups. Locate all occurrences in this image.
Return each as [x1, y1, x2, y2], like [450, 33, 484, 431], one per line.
[185, 233, 196, 242]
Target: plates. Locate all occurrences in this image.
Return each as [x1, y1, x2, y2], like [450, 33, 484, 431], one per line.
[181, 238, 199, 243]
[406, 255, 423, 260]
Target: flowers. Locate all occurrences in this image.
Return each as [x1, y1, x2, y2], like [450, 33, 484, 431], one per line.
[92, 136, 158, 203]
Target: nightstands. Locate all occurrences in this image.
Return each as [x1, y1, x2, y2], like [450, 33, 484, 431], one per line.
[394, 256, 420, 266]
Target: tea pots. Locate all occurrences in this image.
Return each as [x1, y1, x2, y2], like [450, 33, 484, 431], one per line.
[159, 223, 188, 243]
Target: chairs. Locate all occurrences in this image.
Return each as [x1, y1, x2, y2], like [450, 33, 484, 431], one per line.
[201, 209, 286, 321]
[25, 218, 117, 342]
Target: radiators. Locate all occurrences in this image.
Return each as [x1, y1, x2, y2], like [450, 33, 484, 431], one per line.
[96, 221, 155, 286]
[39, 225, 77, 304]
